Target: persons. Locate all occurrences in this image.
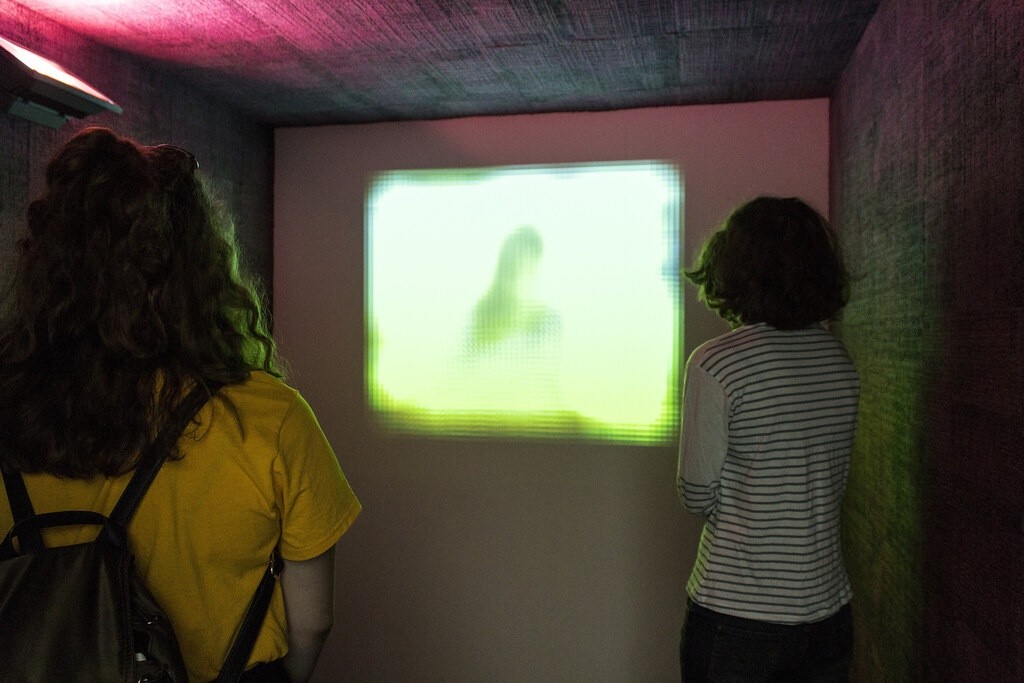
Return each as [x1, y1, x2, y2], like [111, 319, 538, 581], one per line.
[676, 196, 860, 683]
[0, 129, 361, 683]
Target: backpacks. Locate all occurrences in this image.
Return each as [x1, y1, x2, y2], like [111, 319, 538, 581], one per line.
[0, 361, 286, 683]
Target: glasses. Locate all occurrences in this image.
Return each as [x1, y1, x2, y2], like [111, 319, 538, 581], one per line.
[156, 143, 199, 175]
